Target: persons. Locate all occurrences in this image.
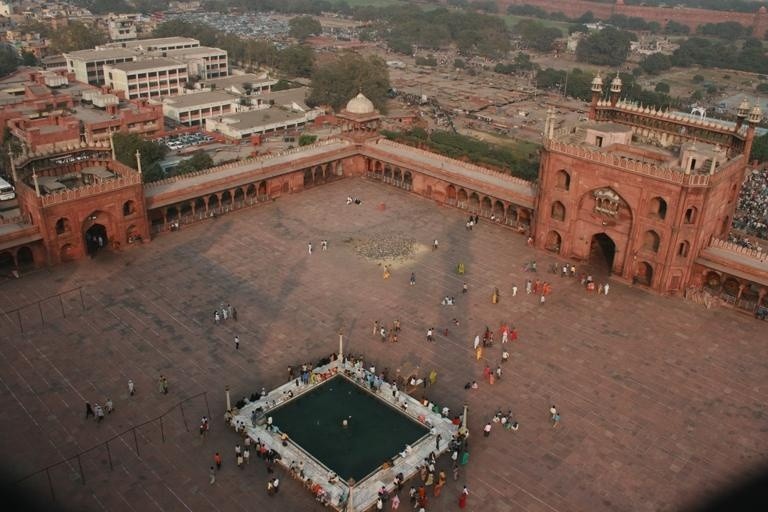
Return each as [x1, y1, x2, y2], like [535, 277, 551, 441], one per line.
[549, 405, 557, 424]
[553, 413, 560, 432]
[88, 234, 103, 249]
[210, 210, 217, 218]
[490, 213, 495, 221]
[434, 239, 439, 248]
[170, 216, 179, 231]
[201, 353, 520, 512]
[324, 240, 328, 251]
[159, 375, 165, 392]
[465, 214, 480, 231]
[527, 236, 534, 248]
[383, 264, 392, 280]
[409, 271, 416, 288]
[521, 256, 611, 305]
[128, 380, 135, 396]
[320, 239, 324, 250]
[212, 304, 239, 350]
[85, 397, 115, 424]
[308, 243, 312, 255]
[371, 283, 520, 385]
[346, 194, 363, 205]
[163, 378, 168, 395]
[730, 168, 768, 249]
